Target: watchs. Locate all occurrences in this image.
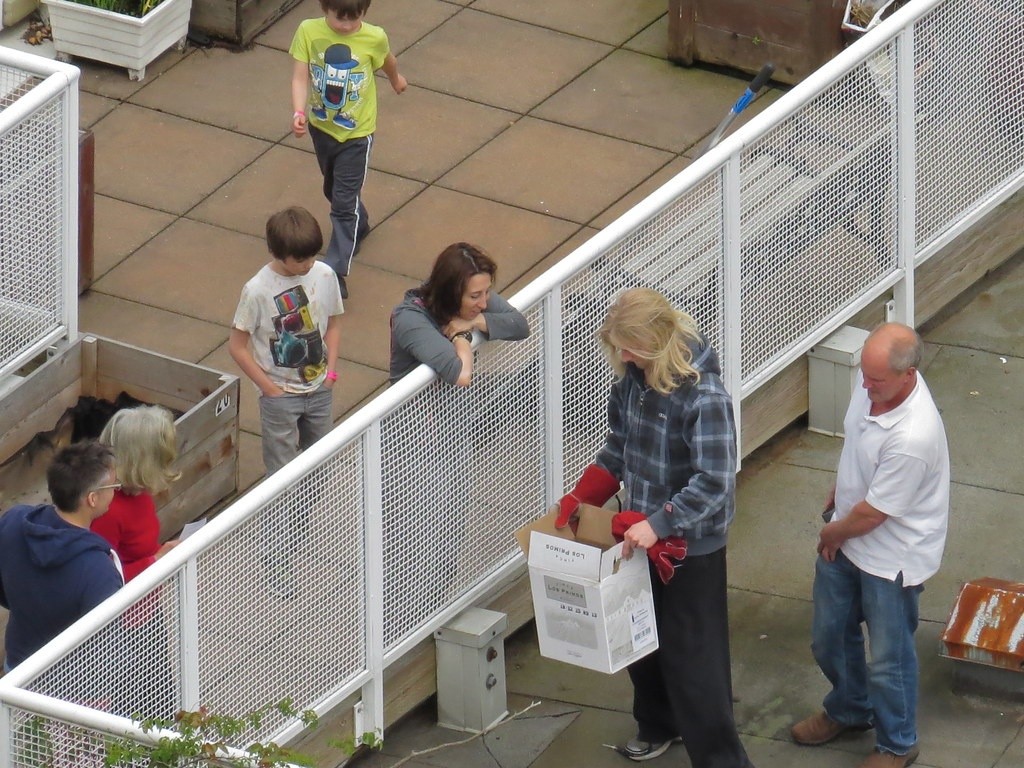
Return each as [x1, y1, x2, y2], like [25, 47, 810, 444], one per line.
[450, 331, 473, 344]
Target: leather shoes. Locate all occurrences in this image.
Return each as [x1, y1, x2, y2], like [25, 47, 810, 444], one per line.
[790, 707, 855, 744]
[861, 738, 917, 768]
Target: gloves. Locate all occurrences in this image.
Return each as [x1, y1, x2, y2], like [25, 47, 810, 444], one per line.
[454, 331, 473, 344]
[609, 510, 687, 584]
[555, 461, 623, 536]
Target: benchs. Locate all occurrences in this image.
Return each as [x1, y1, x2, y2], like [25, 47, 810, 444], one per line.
[521, 116, 892, 405]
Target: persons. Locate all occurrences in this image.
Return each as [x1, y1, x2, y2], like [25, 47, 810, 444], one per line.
[0, 405, 196, 768]
[288, 0, 407, 299]
[389, 242, 530, 389]
[229, 208, 345, 597]
[556, 287, 754, 768]
[793, 320, 950, 768]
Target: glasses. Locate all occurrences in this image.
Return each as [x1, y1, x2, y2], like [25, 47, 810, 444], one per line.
[91, 478, 123, 492]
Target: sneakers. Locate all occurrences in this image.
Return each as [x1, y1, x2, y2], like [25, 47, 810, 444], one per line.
[622, 733, 691, 761]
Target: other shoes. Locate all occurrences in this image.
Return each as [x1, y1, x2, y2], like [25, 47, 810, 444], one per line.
[352, 223, 371, 256]
[337, 275, 349, 299]
[264, 564, 301, 599]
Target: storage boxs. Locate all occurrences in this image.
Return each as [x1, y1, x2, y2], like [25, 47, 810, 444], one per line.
[514, 502, 659, 675]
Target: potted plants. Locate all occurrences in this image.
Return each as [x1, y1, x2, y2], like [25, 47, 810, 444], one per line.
[41, 0, 192, 83]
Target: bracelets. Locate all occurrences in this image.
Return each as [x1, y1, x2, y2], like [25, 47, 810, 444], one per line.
[292, 111, 305, 117]
